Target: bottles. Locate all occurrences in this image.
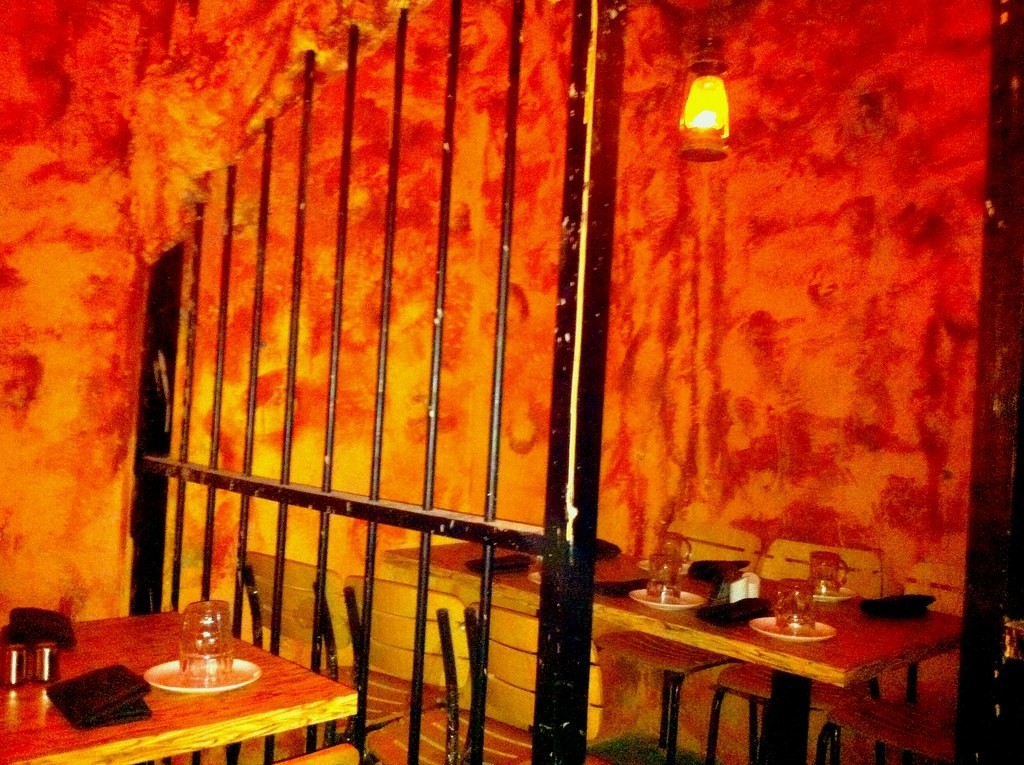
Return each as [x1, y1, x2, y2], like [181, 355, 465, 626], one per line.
[6, 647, 26, 685]
[36, 643, 55, 681]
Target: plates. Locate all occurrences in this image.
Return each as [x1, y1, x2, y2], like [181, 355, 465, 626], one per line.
[144, 658, 261, 692]
[814, 588, 858, 602]
[528, 572, 541, 585]
[750, 618, 836, 643]
[638, 561, 690, 575]
[629, 589, 704, 611]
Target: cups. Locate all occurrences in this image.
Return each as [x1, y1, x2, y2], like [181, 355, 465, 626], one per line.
[775, 579, 817, 636]
[658, 531, 692, 564]
[810, 551, 849, 595]
[647, 553, 683, 604]
[180, 600, 234, 681]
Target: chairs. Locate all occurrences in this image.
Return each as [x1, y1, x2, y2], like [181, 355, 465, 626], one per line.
[241, 518, 966, 765]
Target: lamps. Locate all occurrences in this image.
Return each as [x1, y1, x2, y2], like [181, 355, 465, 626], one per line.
[680, 0, 731, 163]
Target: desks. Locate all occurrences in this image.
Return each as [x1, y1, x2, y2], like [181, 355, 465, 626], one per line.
[0, 609, 359, 765]
[378, 540, 963, 765]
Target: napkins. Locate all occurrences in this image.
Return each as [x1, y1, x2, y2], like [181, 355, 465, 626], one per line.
[464, 553, 532, 575]
[8, 607, 75, 650]
[687, 560, 751, 582]
[594, 578, 655, 597]
[860, 594, 934, 620]
[45, 665, 153, 728]
[699, 598, 770, 627]
[595, 535, 622, 562]
